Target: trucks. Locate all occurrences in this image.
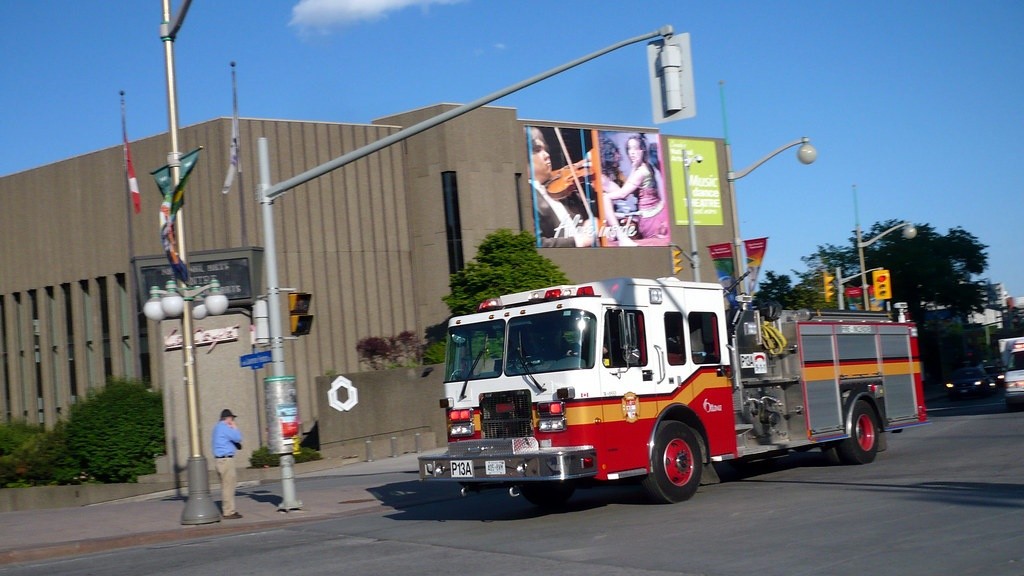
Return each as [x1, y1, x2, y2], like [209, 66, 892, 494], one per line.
[1000, 336, 1024, 408]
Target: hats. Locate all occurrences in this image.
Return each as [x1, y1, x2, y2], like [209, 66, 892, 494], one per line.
[222, 409, 236, 417]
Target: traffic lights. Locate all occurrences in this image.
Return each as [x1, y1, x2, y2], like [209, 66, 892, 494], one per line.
[289, 313, 314, 337]
[823, 270, 836, 302]
[287, 292, 312, 314]
[869, 270, 893, 301]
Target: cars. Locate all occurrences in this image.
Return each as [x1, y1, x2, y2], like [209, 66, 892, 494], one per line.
[944, 361, 1003, 401]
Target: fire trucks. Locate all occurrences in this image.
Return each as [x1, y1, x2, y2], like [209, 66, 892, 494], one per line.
[414, 274, 931, 504]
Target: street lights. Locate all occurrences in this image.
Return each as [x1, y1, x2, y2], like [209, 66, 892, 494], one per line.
[725, 135, 818, 298]
[142, 273, 230, 524]
[855, 222, 919, 311]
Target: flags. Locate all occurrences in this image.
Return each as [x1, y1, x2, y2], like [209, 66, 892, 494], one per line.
[122, 96, 241, 282]
[706, 239, 767, 309]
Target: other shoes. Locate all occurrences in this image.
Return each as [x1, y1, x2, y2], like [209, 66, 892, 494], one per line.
[224, 512, 242, 519]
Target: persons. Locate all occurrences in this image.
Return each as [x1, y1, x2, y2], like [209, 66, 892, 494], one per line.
[567, 328, 610, 369]
[526, 127, 668, 246]
[211, 409, 244, 519]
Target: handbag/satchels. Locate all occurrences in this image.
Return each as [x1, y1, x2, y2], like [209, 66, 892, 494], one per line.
[231, 440, 241, 450]
